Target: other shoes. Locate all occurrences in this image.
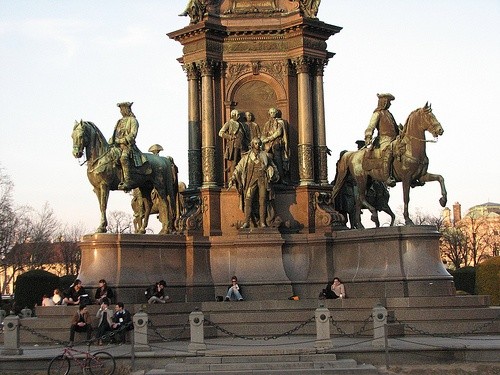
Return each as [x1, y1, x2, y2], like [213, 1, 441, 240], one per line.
[85, 341, 90, 345]
[98, 339, 103, 345]
[94, 339, 99, 345]
[225, 297, 230, 301]
[318, 292, 326, 300]
[67, 342, 73, 347]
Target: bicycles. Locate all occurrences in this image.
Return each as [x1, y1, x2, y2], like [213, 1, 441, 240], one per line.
[47, 339, 116, 375]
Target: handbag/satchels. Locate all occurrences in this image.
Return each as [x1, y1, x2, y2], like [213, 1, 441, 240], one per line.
[79, 294, 92, 305]
[216, 296, 223, 302]
[288, 295, 301, 300]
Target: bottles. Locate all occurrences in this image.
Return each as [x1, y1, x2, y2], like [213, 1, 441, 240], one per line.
[339, 293, 343, 300]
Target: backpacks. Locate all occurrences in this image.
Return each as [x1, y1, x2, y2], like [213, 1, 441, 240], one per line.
[144, 284, 156, 303]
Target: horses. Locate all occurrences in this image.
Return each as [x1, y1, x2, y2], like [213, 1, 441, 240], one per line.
[328, 101, 447, 229]
[71, 118, 182, 234]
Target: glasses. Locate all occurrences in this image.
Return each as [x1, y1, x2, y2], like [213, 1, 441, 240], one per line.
[232, 281, 236, 282]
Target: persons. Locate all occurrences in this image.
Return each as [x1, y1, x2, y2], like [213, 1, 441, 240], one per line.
[225, 276, 244, 301]
[63, 279, 88, 305]
[145, 280, 172, 304]
[42, 289, 62, 306]
[218, 106, 289, 229]
[108, 102, 139, 191]
[95, 279, 114, 305]
[321, 277, 346, 299]
[364, 93, 404, 187]
[331, 150, 355, 229]
[66, 304, 92, 347]
[95, 301, 131, 346]
